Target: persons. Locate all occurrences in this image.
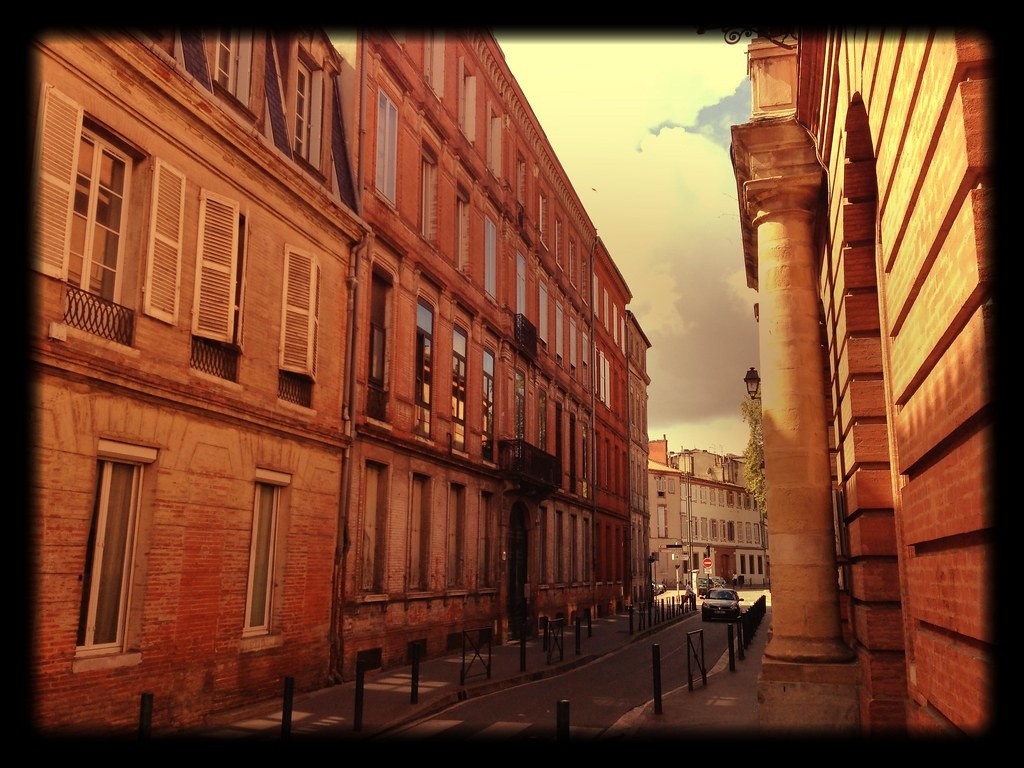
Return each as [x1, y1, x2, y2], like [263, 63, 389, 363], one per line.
[680, 581, 695, 609]
[732, 571, 737, 587]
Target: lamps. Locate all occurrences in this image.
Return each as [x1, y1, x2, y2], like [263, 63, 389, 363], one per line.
[744, 367, 761, 400]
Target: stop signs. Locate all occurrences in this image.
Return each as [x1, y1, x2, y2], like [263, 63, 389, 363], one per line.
[702, 558, 713, 568]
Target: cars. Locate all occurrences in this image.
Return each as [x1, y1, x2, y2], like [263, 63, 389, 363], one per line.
[652, 579, 667, 596]
[700, 588, 744, 621]
